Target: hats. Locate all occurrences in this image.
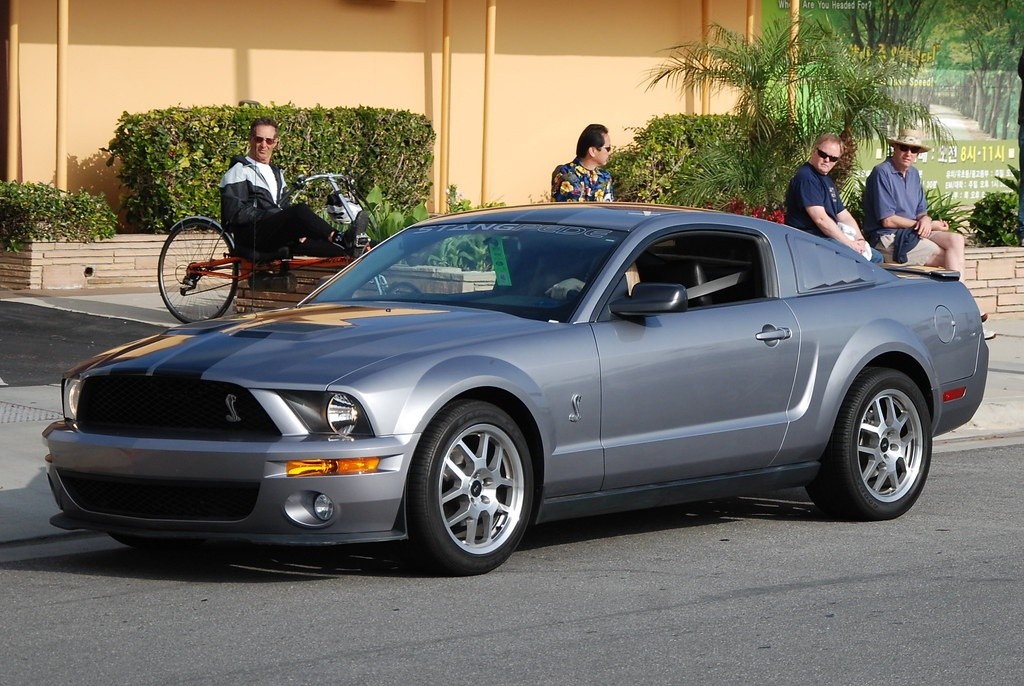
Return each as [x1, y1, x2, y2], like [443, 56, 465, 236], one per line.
[886, 129, 930, 153]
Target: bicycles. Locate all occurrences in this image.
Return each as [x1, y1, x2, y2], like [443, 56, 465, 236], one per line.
[156, 170, 425, 328]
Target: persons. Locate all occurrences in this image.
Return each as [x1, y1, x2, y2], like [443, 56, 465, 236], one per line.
[864, 130, 997, 341]
[530, 246, 640, 298]
[552, 124, 614, 202]
[782, 135, 884, 265]
[220, 116, 369, 258]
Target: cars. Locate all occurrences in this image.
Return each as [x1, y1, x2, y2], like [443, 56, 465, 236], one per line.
[38, 201, 989, 576]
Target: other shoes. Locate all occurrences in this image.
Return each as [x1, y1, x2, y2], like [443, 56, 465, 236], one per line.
[980, 310, 987, 322]
[983, 329, 996, 340]
[332, 211, 369, 258]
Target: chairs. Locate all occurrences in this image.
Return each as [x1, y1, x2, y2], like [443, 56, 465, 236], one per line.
[647, 256, 713, 306]
[510, 243, 588, 296]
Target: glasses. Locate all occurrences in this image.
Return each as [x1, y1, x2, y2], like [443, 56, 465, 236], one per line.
[898, 145, 921, 153]
[816, 147, 840, 162]
[599, 146, 616, 151]
[249, 135, 275, 145]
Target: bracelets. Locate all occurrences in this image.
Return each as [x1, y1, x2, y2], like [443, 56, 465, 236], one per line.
[858, 238, 865, 241]
